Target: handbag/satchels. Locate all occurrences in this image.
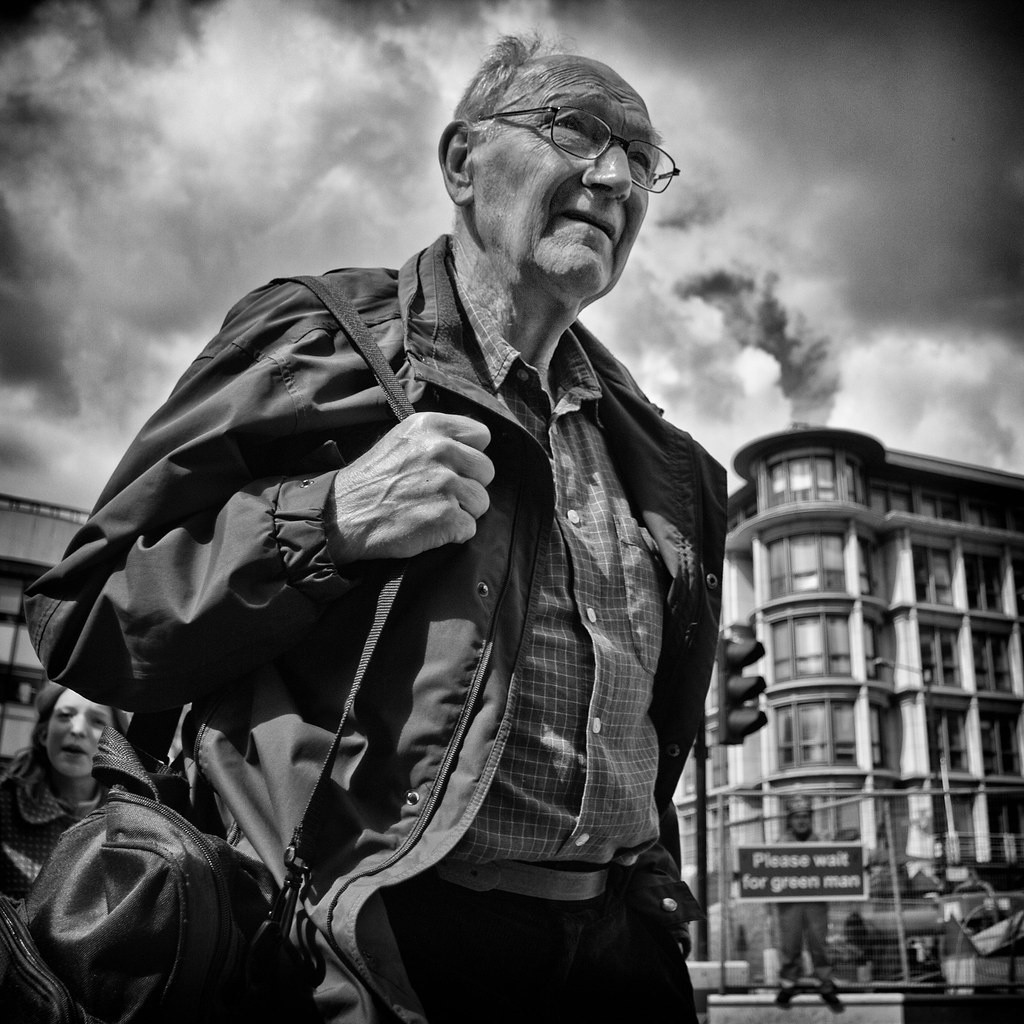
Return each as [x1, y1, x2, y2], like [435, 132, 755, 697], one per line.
[1, 724, 288, 1024]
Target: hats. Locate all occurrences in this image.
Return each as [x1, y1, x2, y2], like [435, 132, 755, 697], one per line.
[785, 797, 814, 815]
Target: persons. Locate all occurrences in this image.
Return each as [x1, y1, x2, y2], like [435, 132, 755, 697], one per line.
[26, 35, 727, 1023]
[732, 800, 871, 1006]
[0, 679, 134, 901]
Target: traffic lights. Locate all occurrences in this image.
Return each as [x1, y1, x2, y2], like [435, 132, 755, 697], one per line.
[719, 637, 769, 745]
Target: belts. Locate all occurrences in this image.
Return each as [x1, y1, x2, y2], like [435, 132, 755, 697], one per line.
[440, 858, 610, 902]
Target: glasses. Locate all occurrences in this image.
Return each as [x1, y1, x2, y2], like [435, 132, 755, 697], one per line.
[477, 106, 681, 194]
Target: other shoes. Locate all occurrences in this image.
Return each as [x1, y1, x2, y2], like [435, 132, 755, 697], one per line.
[822, 994, 841, 1010]
[776, 989, 793, 1005]
